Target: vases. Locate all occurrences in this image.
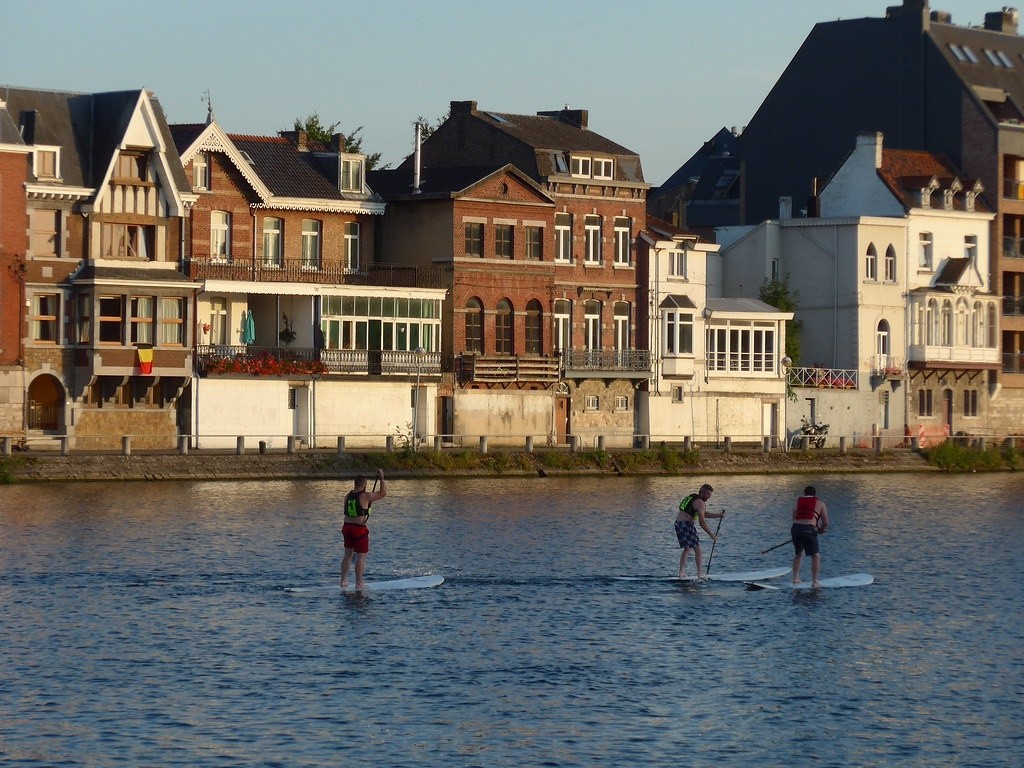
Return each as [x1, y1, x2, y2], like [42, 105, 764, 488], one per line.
[833, 385, 837, 388]
[819, 384, 824, 388]
[847, 385, 851, 389]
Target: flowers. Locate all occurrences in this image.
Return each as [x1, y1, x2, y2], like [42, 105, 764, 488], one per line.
[885, 367, 905, 375]
[198, 353, 329, 377]
[806, 377, 855, 385]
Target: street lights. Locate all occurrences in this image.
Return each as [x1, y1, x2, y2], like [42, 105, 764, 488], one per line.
[411, 345, 427, 454]
[781, 357, 792, 454]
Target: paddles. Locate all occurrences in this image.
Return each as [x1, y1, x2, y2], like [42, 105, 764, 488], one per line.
[761, 529, 818, 554]
[707, 509, 725, 574]
[364, 471, 379, 523]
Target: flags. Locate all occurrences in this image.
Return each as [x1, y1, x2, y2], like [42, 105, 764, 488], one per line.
[138, 348, 153, 376]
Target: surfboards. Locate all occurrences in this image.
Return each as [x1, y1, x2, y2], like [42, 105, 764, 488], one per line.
[612, 567, 793, 582]
[743, 573, 874, 589]
[283, 575, 445, 598]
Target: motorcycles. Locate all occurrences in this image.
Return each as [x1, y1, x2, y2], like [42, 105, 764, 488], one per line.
[792, 415, 830, 450]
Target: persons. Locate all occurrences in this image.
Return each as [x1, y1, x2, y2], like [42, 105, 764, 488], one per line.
[902, 424, 911, 444]
[790, 486, 830, 587]
[674, 484, 726, 579]
[340, 468, 386, 590]
